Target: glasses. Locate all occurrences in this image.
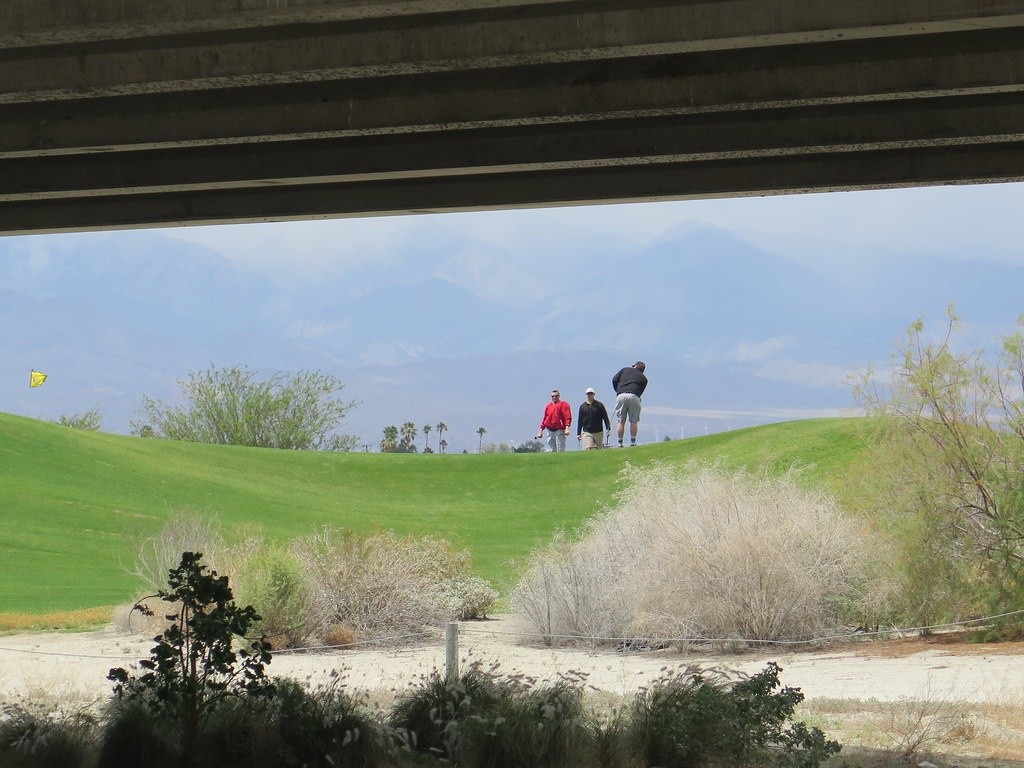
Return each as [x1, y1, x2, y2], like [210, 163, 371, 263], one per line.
[551, 395, 558, 398]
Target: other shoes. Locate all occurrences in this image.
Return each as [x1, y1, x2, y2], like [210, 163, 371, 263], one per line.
[618, 446, 623, 448]
[629, 444, 635, 447]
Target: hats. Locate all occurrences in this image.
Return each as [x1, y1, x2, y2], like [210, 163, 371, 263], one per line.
[585, 388, 596, 394]
[632, 361, 646, 372]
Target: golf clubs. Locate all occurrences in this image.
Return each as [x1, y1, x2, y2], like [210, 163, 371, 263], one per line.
[534, 433, 570, 439]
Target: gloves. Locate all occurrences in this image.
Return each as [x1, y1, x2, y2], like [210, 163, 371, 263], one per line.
[577, 435, 581, 440]
[606, 430, 611, 436]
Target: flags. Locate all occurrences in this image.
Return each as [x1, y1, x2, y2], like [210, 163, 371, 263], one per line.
[30, 372, 48, 388]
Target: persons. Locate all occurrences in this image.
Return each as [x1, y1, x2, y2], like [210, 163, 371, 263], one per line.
[537, 391, 572, 452]
[612, 361, 648, 447]
[577, 388, 611, 451]
